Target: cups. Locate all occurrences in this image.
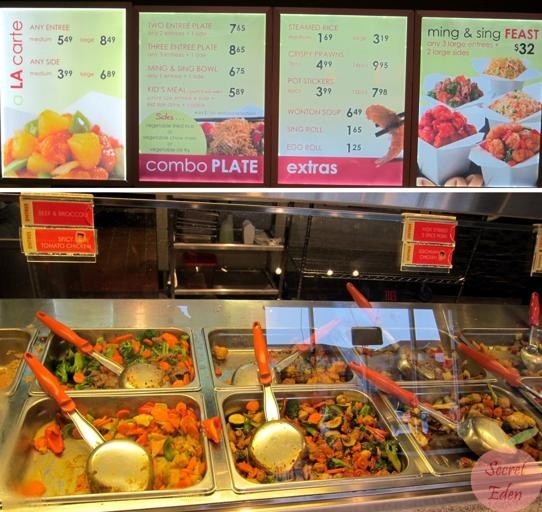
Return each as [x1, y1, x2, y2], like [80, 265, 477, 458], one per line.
[182, 250, 217, 289]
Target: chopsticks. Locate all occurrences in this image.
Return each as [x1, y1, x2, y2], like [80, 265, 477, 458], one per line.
[373, 112, 406, 140]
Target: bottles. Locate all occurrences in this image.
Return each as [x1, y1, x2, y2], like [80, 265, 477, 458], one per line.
[219, 213, 235, 243]
